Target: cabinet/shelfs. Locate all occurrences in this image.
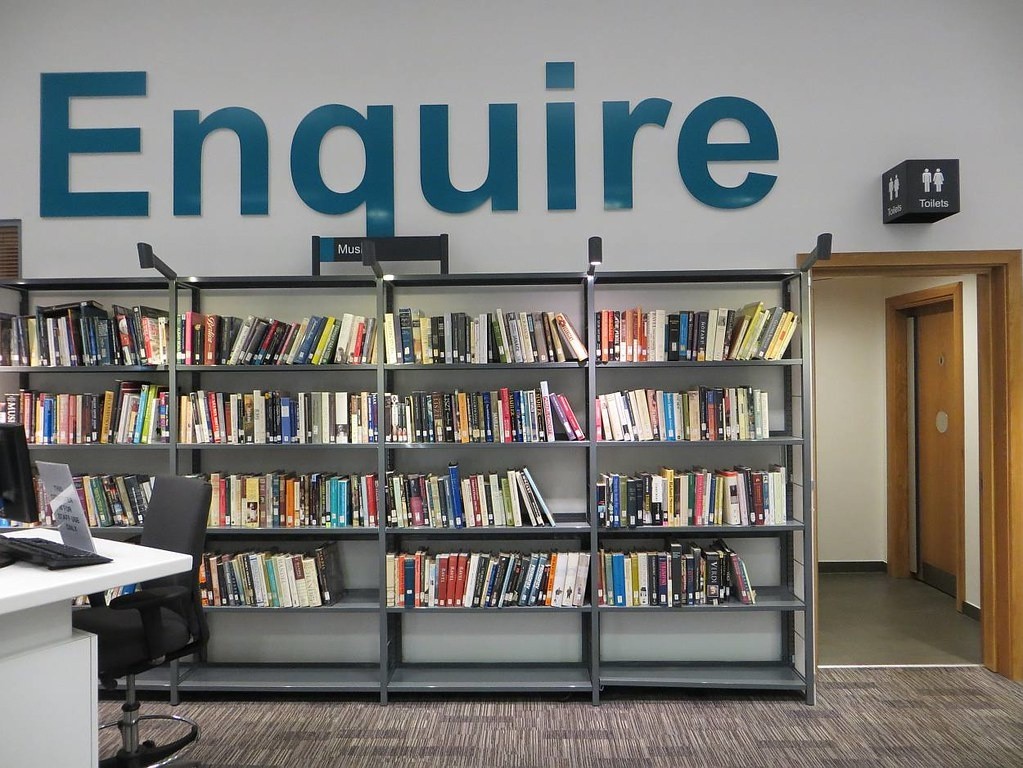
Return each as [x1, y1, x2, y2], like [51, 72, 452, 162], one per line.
[0, 266, 823, 710]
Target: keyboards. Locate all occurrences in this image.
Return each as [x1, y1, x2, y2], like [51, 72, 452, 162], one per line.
[0, 538, 113, 570]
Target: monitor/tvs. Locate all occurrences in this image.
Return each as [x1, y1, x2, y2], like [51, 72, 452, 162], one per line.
[0, 423, 40, 570]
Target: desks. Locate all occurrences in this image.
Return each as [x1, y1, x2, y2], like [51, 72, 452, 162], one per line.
[1, 525, 196, 768]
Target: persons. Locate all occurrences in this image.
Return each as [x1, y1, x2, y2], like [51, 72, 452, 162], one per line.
[565, 586, 573, 601]
[557, 314, 565, 325]
[246, 502, 257, 522]
[554, 586, 563, 604]
[708, 585, 718, 596]
[335, 347, 345, 363]
[576, 585, 582, 603]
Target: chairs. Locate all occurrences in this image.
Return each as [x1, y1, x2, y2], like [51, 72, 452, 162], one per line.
[69, 472, 215, 768]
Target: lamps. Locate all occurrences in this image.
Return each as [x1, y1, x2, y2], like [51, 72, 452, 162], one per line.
[135, 240, 178, 284]
[583, 235, 605, 280]
[798, 232, 834, 274]
[358, 238, 388, 282]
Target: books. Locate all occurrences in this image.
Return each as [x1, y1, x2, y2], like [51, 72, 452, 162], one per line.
[0, 299, 798, 607]
